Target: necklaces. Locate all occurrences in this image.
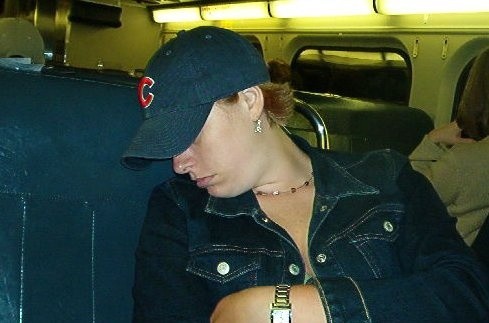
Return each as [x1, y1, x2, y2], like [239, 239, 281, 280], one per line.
[253, 170, 314, 196]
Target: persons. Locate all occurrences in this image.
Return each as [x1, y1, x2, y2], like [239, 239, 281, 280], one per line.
[0, 16, 45, 67]
[408, 48, 489, 246]
[120, 26, 489, 323]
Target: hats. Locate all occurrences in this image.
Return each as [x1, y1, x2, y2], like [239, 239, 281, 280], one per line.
[120, 25, 272, 170]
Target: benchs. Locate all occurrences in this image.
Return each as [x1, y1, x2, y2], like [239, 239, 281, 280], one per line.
[0, 58, 435, 323]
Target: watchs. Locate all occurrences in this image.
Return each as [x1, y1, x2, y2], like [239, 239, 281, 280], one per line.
[270, 283, 292, 323]
